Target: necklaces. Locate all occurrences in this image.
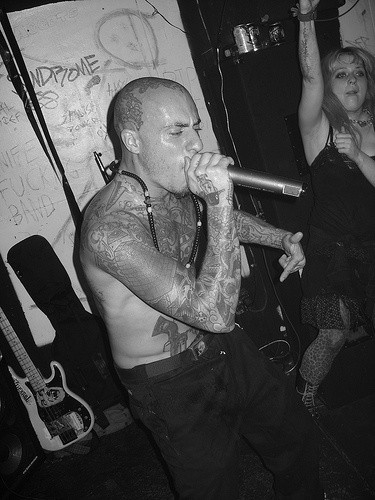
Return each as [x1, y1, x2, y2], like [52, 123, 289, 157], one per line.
[349, 108, 374, 128]
[116, 169, 203, 268]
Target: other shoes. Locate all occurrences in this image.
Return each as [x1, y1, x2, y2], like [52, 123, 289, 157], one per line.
[295, 373, 320, 423]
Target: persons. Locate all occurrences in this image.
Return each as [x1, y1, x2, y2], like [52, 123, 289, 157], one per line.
[78, 77, 326, 500]
[294, 0, 375, 419]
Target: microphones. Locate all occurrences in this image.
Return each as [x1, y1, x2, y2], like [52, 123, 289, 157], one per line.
[227, 164, 307, 197]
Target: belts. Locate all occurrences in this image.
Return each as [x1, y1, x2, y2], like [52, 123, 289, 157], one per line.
[145, 335, 213, 378]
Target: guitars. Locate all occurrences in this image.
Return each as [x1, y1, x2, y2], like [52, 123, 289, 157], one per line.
[0, 307, 95, 452]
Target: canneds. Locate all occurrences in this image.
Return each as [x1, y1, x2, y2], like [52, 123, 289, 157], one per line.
[233, 23, 254, 54]
[245, 22, 263, 53]
[267, 22, 286, 49]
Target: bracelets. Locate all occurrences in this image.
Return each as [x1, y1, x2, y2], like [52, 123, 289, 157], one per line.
[297, 10, 317, 21]
